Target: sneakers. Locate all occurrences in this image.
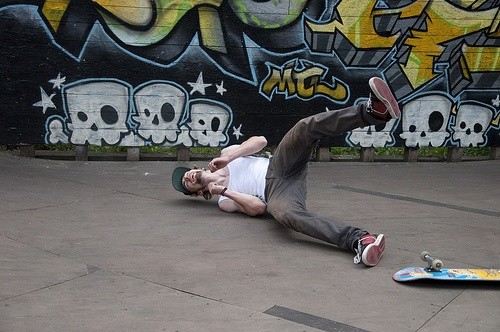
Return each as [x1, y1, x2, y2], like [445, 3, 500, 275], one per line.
[366, 77, 401, 121]
[353, 233, 386, 266]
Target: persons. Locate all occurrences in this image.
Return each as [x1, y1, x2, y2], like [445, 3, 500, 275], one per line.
[171, 77, 399, 266]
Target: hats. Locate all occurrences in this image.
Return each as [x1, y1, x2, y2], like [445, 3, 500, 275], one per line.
[172, 167, 192, 194]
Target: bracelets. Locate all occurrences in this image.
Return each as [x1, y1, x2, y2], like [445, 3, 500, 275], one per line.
[221, 188, 227, 196]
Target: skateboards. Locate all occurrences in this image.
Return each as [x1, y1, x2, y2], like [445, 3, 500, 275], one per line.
[392, 250, 500, 282]
